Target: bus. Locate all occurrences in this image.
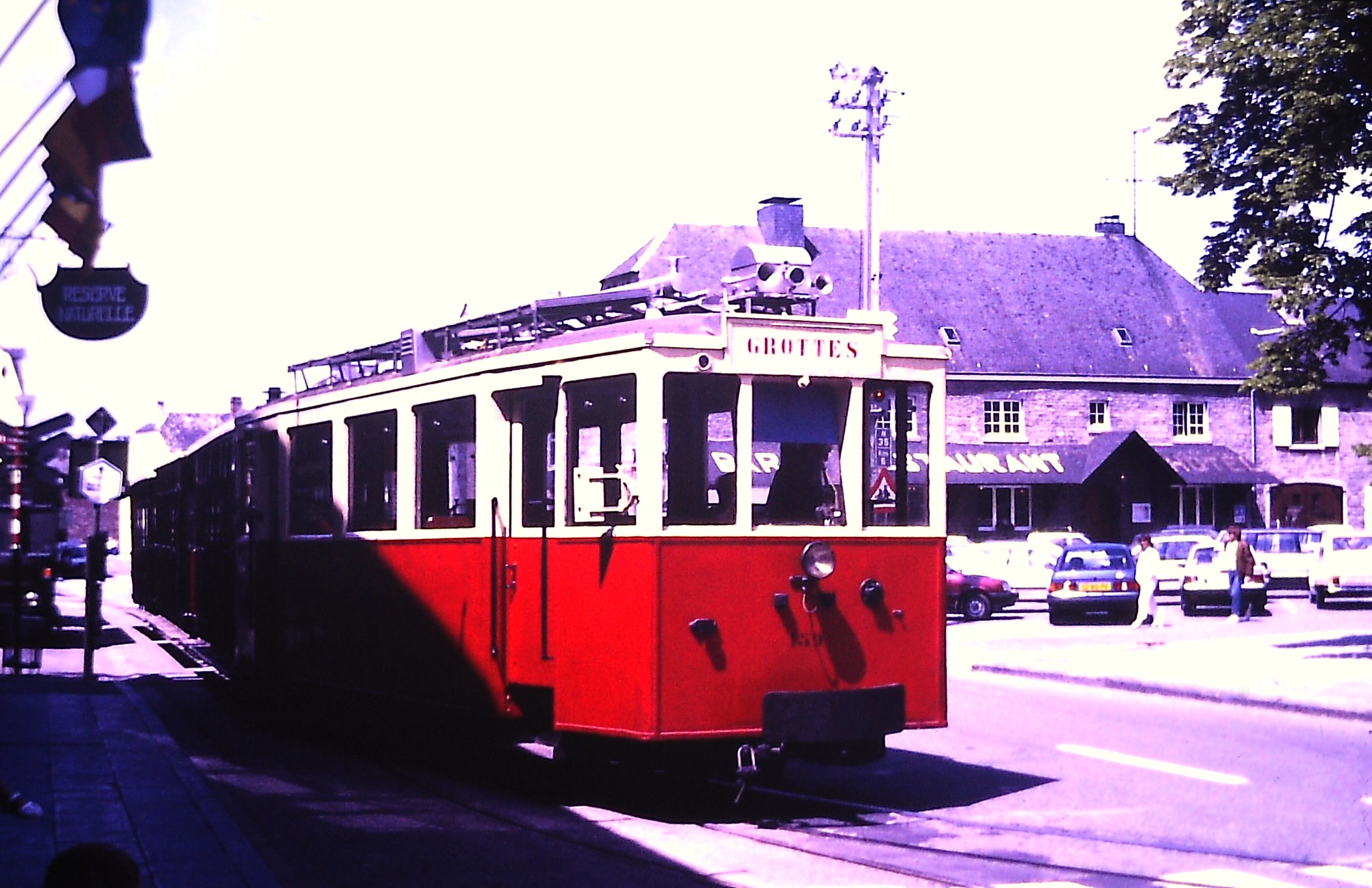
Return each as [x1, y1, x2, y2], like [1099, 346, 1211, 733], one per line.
[128, 242, 948, 805]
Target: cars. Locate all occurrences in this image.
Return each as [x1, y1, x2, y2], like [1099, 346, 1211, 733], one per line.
[946, 520, 1372, 624]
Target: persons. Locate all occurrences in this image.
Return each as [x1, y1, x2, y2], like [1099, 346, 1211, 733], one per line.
[41, 840, 143, 888]
[1224, 524, 1256, 625]
[765, 439, 836, 524]
[702, 471, 754, 528]
[1129, 533, 1166, 628]
